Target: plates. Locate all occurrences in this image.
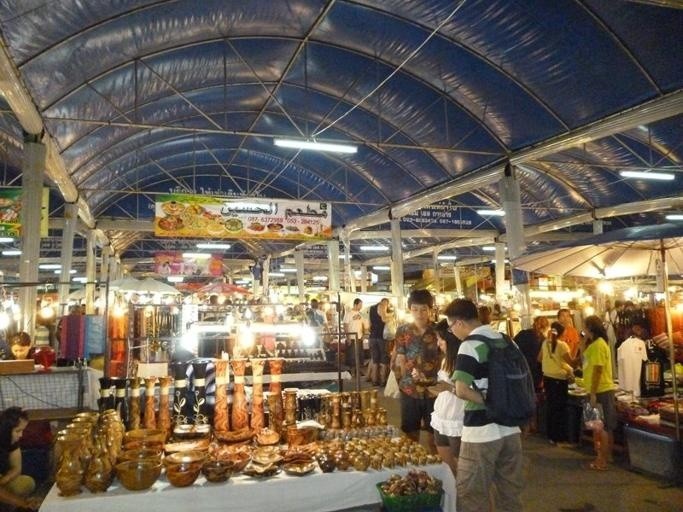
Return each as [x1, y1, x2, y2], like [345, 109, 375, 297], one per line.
[159, 200, 313, 237]
[238, 430, 316, 481]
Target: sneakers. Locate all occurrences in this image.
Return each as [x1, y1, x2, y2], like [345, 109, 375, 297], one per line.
[366, 377, 385, 386]
[557, 441, 578, 449]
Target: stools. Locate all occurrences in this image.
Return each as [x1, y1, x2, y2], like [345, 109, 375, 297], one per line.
[577, 405, 600, 455]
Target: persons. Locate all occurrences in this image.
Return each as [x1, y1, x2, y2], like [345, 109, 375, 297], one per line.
[207, 295, 332, 338]
[9, 332, 33, 359]
[0, 407, 38, 510]
[342, 297, 395, 387]
[392, 289, 521, 510]
[511, 307, 648, 469]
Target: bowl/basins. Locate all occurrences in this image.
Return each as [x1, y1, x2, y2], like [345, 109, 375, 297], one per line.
[114, 426, 252, 491]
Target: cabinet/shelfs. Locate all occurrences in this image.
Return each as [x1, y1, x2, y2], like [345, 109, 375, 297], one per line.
[125, 302, 353, 391]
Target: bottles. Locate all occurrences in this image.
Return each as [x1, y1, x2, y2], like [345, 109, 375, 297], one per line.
[55, 409, 125, 499]
[314, 437, 446, 474]
[298, 388, 391, 434]
[95, 375, 173, 444]
[173, 356, 298, 440]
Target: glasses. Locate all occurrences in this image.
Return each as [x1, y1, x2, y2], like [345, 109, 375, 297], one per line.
[446, 321, 456, 333]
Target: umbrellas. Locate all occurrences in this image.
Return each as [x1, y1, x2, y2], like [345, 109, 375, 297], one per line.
[511, 216, 683, 440]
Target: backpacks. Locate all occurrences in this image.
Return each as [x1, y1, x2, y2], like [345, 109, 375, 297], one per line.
[463, 333, 536, 427]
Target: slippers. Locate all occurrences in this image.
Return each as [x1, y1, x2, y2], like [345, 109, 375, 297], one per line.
[589, 462, 608, 471]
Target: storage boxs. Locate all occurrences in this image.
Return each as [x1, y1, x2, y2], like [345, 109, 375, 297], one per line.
[622, 425, 679, 480]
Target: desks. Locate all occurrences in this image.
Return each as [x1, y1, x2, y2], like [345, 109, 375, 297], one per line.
[0, 364, 101, 423]
[39, 436, 458, 512]
